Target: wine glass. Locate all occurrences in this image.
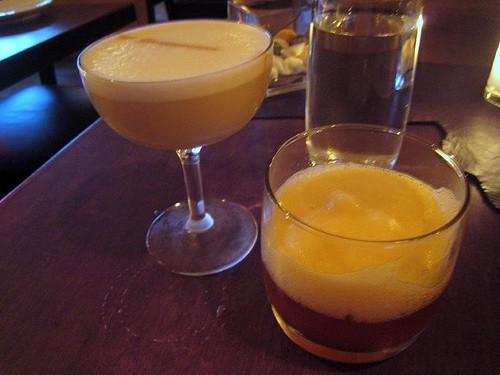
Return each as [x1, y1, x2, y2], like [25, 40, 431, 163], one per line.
[74, 18, 275, 274]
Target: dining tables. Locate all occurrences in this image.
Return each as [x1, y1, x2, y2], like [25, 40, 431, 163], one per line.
[0, 47, 500, 375]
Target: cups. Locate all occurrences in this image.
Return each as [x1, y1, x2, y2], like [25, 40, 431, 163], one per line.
[255, 124, 475, 365]
[306, 0, 426, 171]
